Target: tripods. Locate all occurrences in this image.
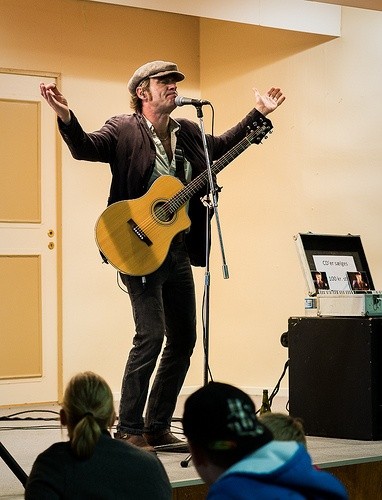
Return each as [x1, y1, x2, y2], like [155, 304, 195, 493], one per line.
[154, 100, 229, 468]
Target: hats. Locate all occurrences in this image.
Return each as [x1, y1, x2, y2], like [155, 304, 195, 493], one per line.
[128, 60, 186, 95]
[182, 380, 274, 441]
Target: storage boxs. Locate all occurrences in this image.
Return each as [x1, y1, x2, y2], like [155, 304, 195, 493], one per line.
[294, 233, 382, 318]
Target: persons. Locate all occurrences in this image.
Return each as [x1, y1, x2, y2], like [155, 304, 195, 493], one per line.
[40, 60, 286, 453]
[24, 371, 172, 500]
[182, 381, 347, 500]
[258, 412, 307, 450]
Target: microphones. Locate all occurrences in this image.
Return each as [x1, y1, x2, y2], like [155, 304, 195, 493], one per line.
[174, 95, 210, 107]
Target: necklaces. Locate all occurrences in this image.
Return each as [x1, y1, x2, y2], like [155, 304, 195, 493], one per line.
[160, 136, 169, 141]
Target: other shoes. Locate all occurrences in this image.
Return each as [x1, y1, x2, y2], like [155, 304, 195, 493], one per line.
[117, 433, 157, 456]
[146, 431, 191, 452]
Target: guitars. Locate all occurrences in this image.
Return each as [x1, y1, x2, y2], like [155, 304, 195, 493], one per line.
[94, 115, 274, 277]
